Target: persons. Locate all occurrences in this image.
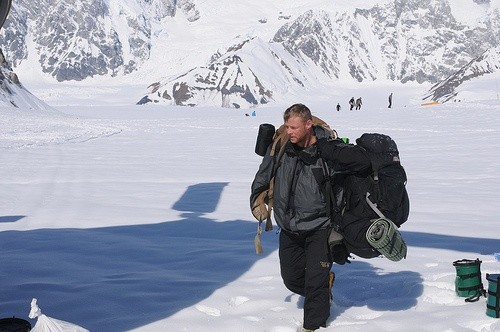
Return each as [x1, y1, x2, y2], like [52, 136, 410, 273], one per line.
[388, 93, 393, 108]
[356, 97, 362, 110]
[336, 103, 340, 111]
[250, 103, 345, 331]
[349, 97, 355, 111]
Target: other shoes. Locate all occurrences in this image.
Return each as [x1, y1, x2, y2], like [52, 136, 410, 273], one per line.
[301, 328, 314, 332]
[328, 271, 335, 303]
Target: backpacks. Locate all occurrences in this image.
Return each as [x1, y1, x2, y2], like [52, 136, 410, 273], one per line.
[318, 133, 410, 265]
